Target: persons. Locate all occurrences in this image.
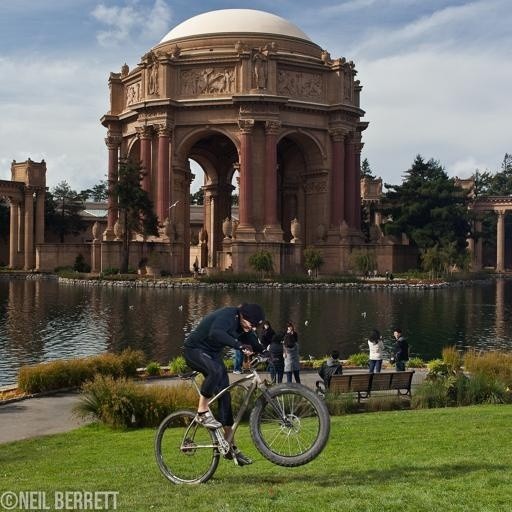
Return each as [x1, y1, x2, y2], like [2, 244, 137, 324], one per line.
[389, 328, 409, 371]
[183, 304, 273, 465]
[367, 329, 384, 374]
[233, 302, 301, 384]
[318, 350, 343, 388]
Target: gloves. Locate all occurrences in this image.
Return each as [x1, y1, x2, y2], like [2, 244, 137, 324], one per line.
[262, 349, 273, 361]
[234, 342, 255, 356]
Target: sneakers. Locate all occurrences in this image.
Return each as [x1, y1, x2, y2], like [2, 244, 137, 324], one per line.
[221, 444, 251, 466]
[233, 366, 248, 375]
[195, 411, 221, 429]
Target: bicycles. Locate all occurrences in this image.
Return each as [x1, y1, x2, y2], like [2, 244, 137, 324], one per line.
[154, 344, 331, 484]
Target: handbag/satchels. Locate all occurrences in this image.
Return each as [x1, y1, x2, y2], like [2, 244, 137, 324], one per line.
[390, 353, 397, 365]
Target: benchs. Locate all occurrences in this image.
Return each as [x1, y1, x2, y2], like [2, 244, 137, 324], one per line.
[315, 370, 416, 404]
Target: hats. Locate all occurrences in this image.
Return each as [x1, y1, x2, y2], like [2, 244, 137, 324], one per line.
[241, 302, 265, 326]
